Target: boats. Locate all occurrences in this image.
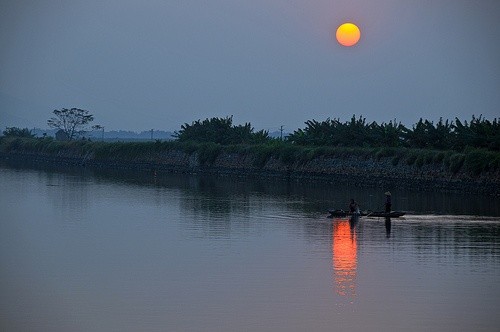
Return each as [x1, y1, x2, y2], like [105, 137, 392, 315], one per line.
[326, 209, 406, 217]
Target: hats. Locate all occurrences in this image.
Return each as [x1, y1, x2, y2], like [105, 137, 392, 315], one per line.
[385, 191, 392, 196]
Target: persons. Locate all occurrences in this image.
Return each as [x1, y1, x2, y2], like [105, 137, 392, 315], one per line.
[385, 217, 392, 239]
[348, 198, 360, 212]
[383, 191, 393, 213]
[348, 216, 360, 229]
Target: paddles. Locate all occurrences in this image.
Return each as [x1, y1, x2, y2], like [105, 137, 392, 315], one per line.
[366, 202, 385, 217]
